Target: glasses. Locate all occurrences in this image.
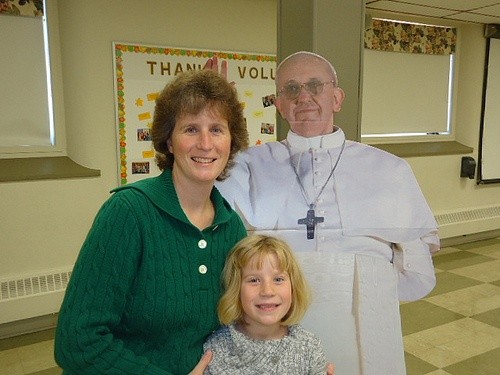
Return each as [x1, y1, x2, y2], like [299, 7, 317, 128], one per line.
[277, 80, 335, 99]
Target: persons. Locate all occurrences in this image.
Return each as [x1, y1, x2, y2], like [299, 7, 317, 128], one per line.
[52, 69, 335, 375]
[216, 51, 442, 375]
[202, 234, 328, 375]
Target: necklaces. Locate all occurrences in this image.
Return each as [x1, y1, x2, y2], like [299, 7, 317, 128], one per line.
[284, 138, 347, 240]
